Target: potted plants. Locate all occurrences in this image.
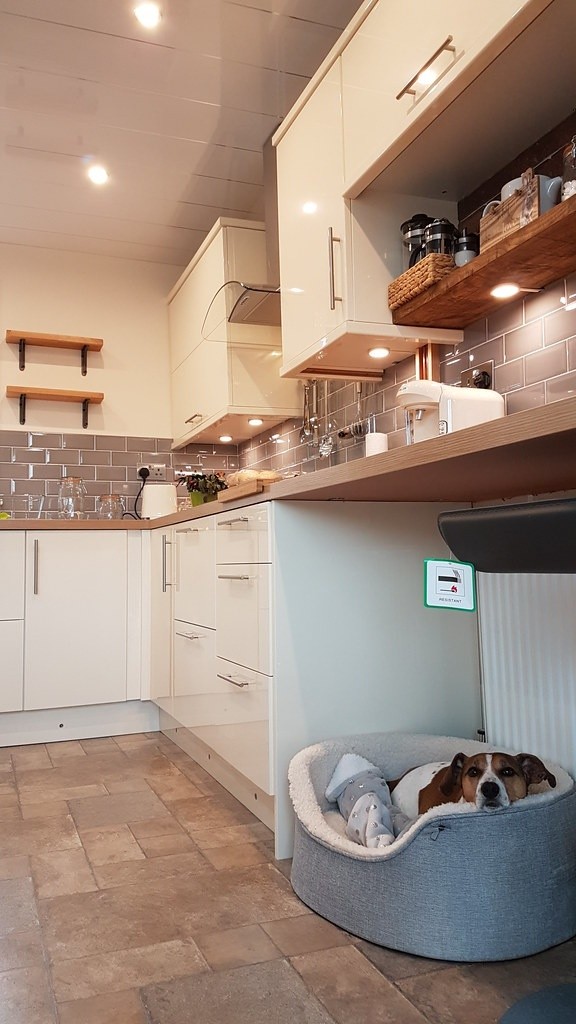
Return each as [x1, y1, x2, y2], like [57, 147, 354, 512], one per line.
[174, 472, 228, 507]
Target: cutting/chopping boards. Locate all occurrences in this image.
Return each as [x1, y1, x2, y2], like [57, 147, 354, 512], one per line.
[217, 478, 285, 503]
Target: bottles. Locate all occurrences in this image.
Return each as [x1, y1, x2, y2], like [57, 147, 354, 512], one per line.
[96, 494, 124, 519]
[560, 134, 576, 202]
[59, 476, 87, 518]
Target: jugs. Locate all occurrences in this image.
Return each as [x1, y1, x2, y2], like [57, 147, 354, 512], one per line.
[423, 217, 459, 261]
[482, 174, 563, 218]
[399, 212, 441, 274]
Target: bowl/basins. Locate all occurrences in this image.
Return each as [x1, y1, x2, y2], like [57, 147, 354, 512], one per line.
[454, 250, 477, 267]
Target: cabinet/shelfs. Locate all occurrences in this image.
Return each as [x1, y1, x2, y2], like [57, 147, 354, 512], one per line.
[0, 529, 160, 747]
[168, 214, 305, 452]
[6, 329, 105, 430]
[340, 0, 576, 200]
[149, 500, 484, 860]
[270, 0, 463, 382]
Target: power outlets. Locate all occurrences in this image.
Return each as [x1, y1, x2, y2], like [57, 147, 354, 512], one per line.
[174, 464, 203, 481]
[460, 359, 494, 391]
[137, 462, 167, 481]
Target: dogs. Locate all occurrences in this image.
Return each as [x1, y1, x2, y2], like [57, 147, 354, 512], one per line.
[386, 752, 558, 813]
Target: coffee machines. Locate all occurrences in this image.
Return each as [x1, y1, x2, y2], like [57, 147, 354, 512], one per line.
[396, 379, 506, 456]
